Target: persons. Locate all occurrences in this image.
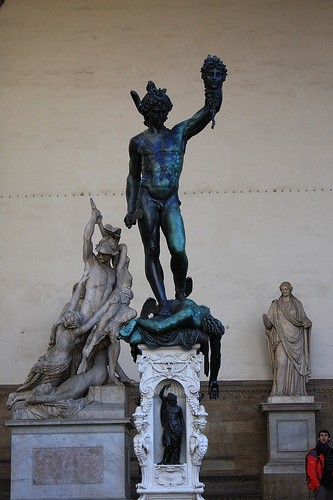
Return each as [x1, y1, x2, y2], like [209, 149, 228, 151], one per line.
[263, 282, 312, 397]
[305, 429, 333, 500]
[201, 54, 228, 130]
[158, 383, 185, 465]
[124, 80, 223, 304]
[8, 190, 138, 421]
[119, 298, 224, 401]
